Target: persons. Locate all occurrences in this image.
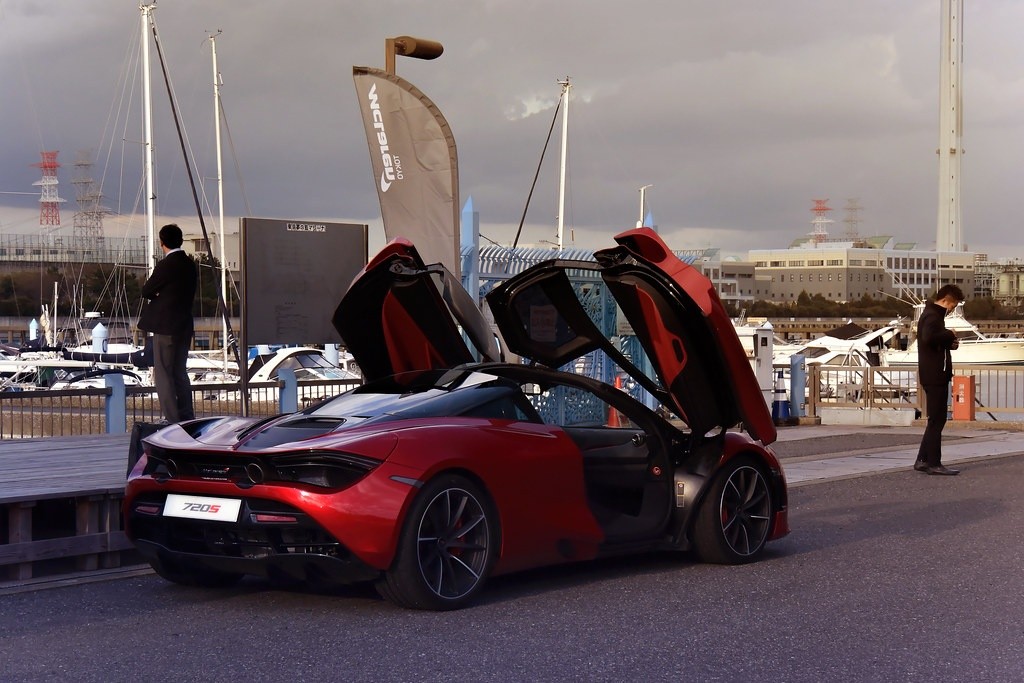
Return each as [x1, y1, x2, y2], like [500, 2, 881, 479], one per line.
[136, 223, 199, 425]
[913, 283, 965, 475]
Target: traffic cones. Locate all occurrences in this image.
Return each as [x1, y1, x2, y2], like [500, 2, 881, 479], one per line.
[602, 375, 632, 430]
[771, 371, 799, 427]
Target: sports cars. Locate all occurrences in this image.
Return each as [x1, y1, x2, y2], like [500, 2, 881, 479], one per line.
[115, 227, 790, 611]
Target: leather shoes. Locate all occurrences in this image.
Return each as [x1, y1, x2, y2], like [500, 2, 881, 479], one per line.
[914, 460, 928, 472]
[927, 464, 961, 475]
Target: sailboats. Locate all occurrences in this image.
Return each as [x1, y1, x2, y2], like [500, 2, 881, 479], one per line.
[2, 2, 1023, 394]
